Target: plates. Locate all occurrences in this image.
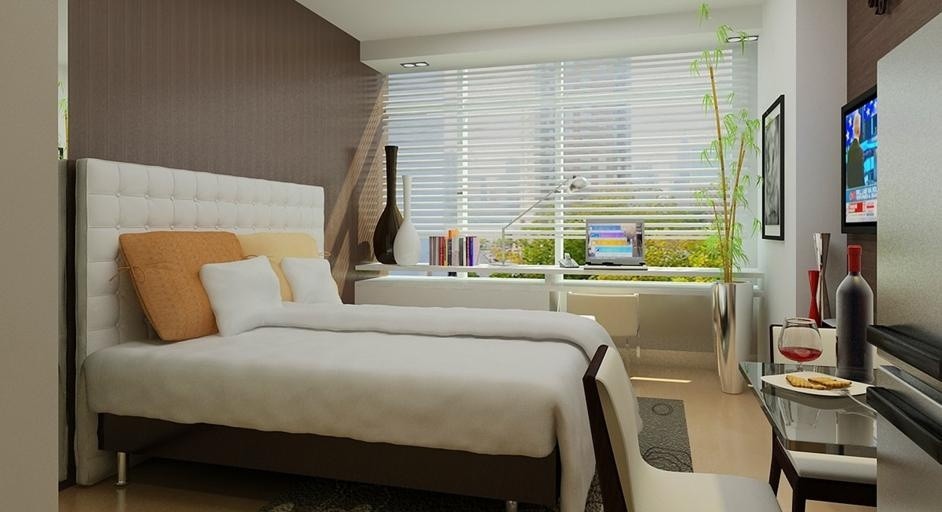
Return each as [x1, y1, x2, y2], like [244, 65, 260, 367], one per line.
[761, 370, 876, 397]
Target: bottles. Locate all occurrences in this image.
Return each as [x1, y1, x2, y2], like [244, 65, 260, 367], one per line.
[835, 245, 876, 386]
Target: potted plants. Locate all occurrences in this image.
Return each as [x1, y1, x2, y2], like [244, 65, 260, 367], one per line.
[686, 0, 775, 396]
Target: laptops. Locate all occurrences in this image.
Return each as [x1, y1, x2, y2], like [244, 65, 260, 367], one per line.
[584, 219, 648, 271]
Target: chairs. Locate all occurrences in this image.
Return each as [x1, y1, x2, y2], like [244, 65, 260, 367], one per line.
[582, 344, 783, 512]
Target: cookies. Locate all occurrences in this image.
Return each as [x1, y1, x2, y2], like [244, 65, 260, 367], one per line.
[786, 375, 830, 390]
[808, 377, 852, 387]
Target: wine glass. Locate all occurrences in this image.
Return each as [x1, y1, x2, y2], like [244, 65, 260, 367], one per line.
[780, 315, 821, 383]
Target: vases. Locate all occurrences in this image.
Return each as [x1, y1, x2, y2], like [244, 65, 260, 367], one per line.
[372, 144, 424, 267]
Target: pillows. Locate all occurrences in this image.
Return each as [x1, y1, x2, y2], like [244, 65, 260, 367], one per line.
[117, 231, 344, 342]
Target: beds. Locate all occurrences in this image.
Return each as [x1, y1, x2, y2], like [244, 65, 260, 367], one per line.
[75, 156, 642, 512]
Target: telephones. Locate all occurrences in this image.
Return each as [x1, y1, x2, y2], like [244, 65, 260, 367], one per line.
[558, 253, 578, 268]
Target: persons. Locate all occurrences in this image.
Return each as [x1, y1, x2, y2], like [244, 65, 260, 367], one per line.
[848, 113, 865, 187]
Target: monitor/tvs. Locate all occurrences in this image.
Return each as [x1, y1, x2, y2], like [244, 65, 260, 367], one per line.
[841, 83, 877, 236]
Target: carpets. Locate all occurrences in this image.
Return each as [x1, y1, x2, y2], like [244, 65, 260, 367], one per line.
[254, 397, 693, 511]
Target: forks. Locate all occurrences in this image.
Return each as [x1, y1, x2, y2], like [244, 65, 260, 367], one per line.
[835, 386, 880, 417]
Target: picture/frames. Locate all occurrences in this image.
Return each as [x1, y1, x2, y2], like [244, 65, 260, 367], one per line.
[760, 94, 786, 239]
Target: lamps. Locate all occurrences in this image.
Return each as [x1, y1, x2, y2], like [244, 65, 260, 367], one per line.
[488, 174, 590, 267]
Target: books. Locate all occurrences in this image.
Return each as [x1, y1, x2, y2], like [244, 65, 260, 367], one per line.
[429, 229, 477, 266]
